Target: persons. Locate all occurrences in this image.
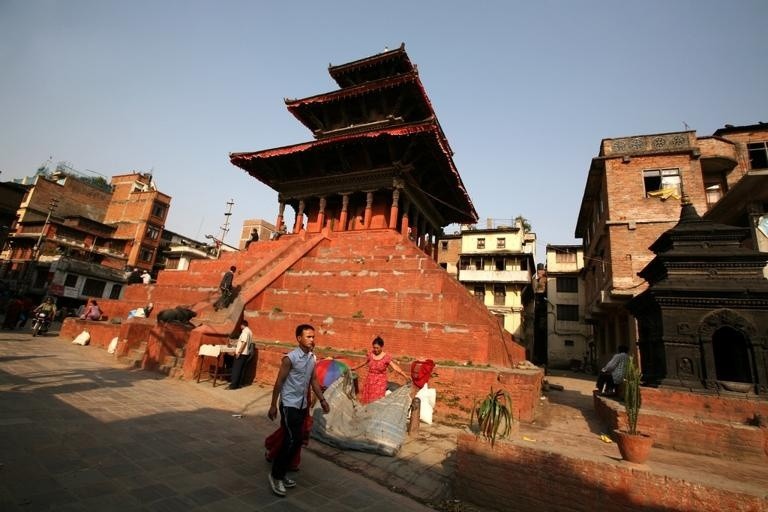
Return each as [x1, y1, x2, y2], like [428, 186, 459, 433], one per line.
[220, 266, 237, 308]
[592, 344, 632, 398]
[268, 325, 329, 496]
[140, 269, 152, 284]
[226, 320, 252, 389]
[270, 222, 287, 241]
[33, 296, 57, 321]
[80, 297, 103, 320]
[292, 224, 305, 233]
[244, 229, 258, 250]
[349, 337, 413, 405]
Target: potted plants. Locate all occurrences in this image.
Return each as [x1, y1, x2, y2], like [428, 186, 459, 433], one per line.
[615, 346, 654, 462]
[471, 382, 516, 445]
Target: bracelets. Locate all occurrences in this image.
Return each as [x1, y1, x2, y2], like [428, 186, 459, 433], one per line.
[320, 399, 325, 403]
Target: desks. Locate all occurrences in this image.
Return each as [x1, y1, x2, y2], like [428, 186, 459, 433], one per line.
[197, 345, 239, 387]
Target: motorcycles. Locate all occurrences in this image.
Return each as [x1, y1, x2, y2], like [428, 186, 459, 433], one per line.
[30, 309, 55, 337]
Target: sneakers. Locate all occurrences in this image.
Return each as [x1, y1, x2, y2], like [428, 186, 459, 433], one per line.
[268, 472, 297, 495]
